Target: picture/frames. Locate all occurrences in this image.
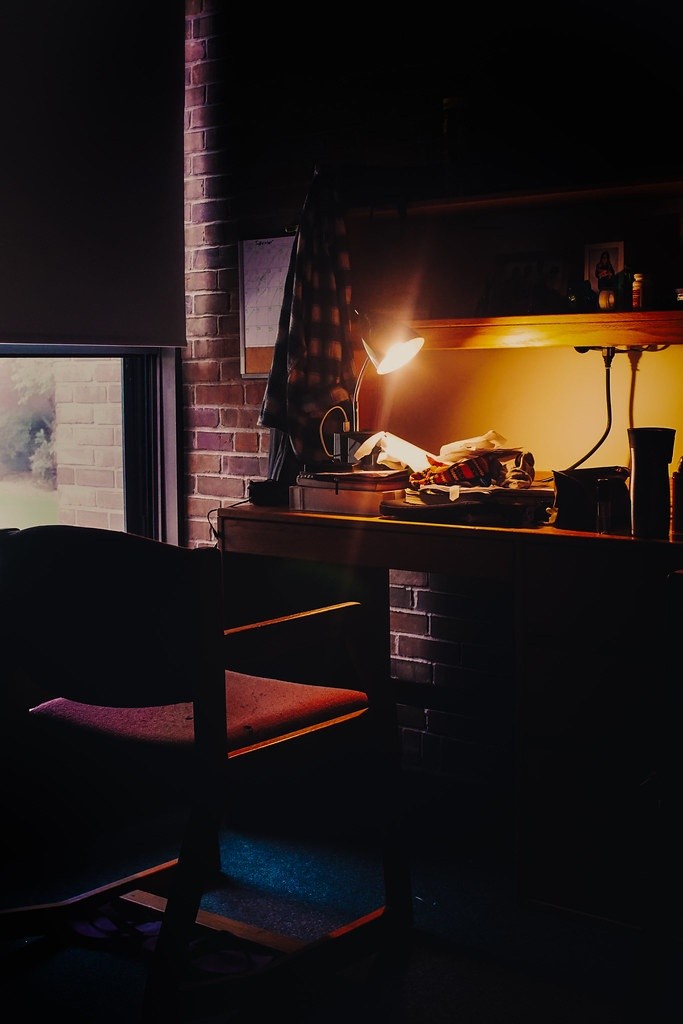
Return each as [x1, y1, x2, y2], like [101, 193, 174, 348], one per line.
[584, 240, 632, 309]
[489, 250, 569, 313]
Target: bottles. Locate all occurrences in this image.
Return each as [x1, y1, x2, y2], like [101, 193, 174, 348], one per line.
[627, 427, 672, 538]
[672, 457, 683, 536]
[599, 284, 616, 311]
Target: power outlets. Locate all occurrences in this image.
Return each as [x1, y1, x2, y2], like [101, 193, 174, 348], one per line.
[332, 432, 374, 466]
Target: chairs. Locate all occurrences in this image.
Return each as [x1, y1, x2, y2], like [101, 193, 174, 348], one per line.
[0, 524, 415, 1024]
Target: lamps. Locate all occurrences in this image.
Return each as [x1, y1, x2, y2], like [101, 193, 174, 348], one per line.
[311, 308, 425, 471]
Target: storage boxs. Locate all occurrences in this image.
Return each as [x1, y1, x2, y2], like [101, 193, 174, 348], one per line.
[288, 486, 405, 516]
